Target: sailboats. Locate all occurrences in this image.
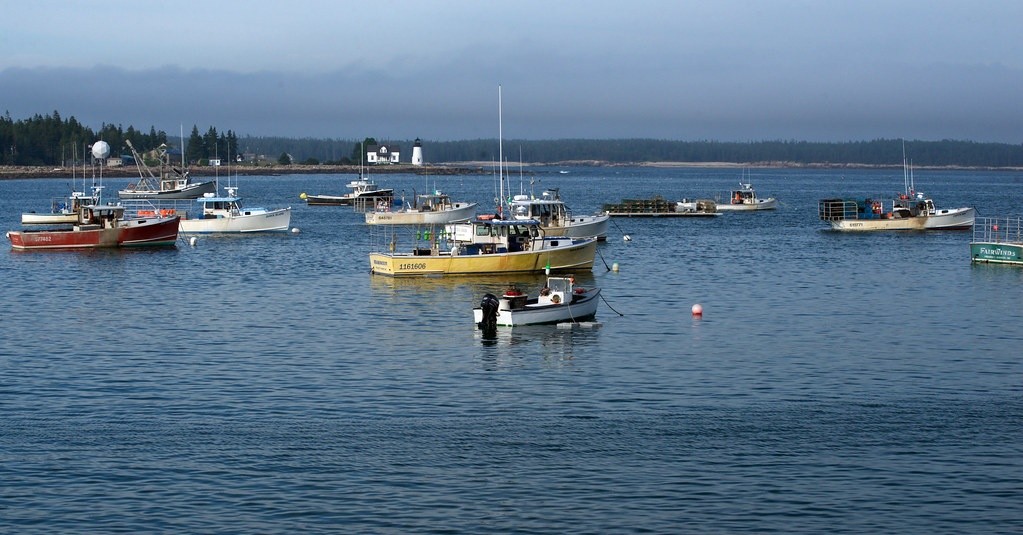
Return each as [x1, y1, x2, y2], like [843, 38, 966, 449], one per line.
[306, 143, 394, 206]
[365, 83, 610, 279]
[818, 136, 975, 233]
[6, 134, 292, 252]
[117, 123, 215, 199]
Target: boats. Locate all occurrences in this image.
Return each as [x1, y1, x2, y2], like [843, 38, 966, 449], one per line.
[472, 265, 602, 327]
[603, 180, 778, 216]
[971, 216, 1023, 266]
